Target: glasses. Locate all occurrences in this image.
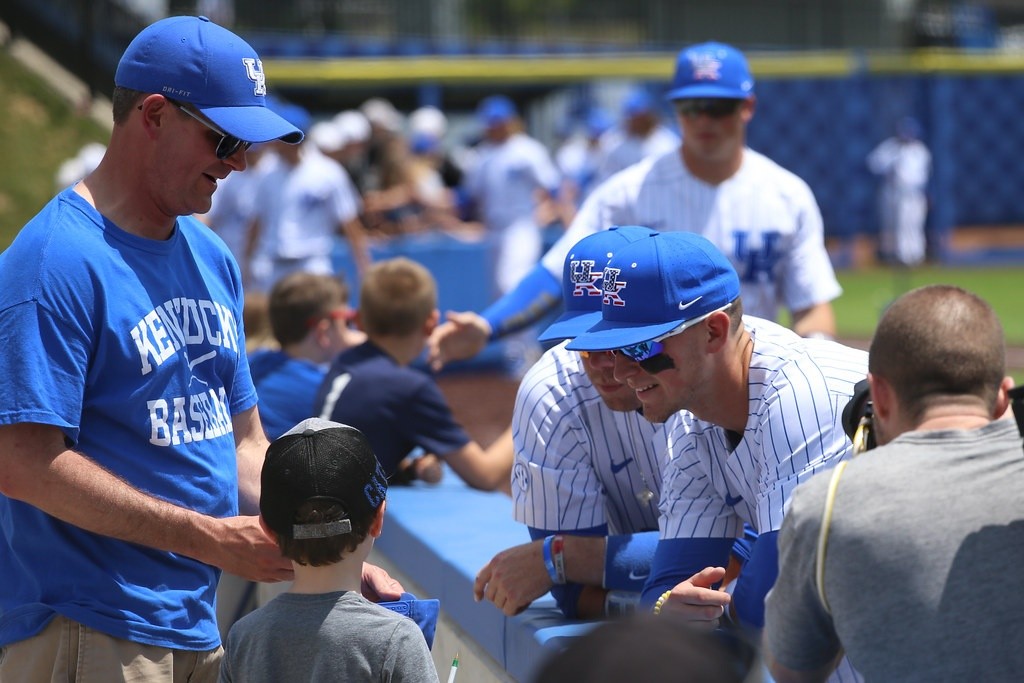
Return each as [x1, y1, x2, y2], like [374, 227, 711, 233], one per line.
[578, 350, 617, 360]
[609, 303, 733, 363]
[328, 307, 357, 325]
[137, 96, 252, 159]
[673, 98, 747, 120]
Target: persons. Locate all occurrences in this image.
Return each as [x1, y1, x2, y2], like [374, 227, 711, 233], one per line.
[425, 39, 843, 374]
[247, 270, 350, 440]
[865, 116, 935, 262]
[212, 418, 442, 683]
[311, 256, 513, 495]
[1, 15, 406, 683]
[764, 285, 1024, 683]
[566, 229, 874, 683]
[57, 85, 682, 354]
[474, 224, 679, 623]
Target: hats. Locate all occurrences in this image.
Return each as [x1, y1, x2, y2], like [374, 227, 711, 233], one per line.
[115, 16, 305, 144]
[666, 42, 756, 100]
[566, 230, 743, 350]
[536, 224, 656, 343]
[476, 86, 652, 138]
[261, 417, 391, 540]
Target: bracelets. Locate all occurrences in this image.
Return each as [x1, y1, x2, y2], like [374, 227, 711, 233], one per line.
[651, 589, 674, 617]
[541, 533, 565, 587]
